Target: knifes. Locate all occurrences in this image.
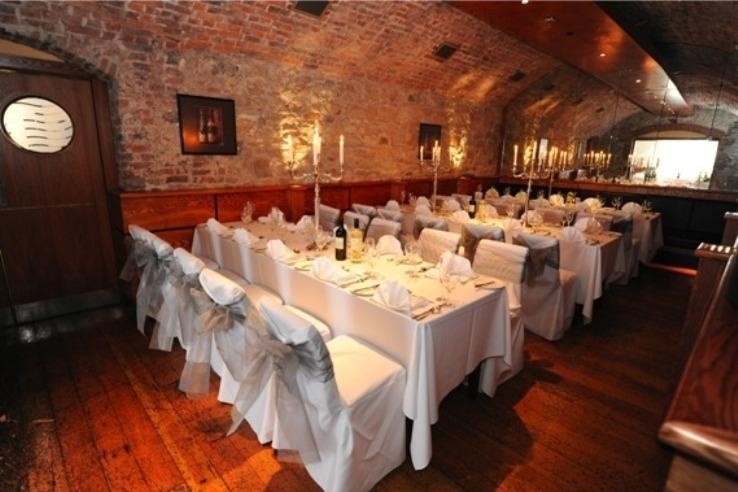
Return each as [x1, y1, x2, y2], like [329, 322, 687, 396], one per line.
[353, 284, 380, 293]
[340, 276, 368, 289]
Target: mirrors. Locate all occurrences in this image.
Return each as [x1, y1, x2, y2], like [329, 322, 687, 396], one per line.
[499, 41, 738, 195]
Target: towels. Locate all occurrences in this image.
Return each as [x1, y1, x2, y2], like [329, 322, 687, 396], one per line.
[233, 227, 258, 246]
[268, 206, 285, 222]
[264, 239, 296, 261]
[207, 217, 227, 234]
[294, 215, 313, 230]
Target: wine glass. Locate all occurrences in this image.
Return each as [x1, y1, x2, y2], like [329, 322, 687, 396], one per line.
[241, 199, 329, 262]
[364, 236, 469, 311]
[503, 187, 655, 240]
[400, 189, 406, 210]
[408, 192, 416, 207]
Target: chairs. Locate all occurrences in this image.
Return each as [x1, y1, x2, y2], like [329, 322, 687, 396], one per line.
[125, 222, 219, 338]
[167, 247, 282, 377]
[256, 297, 406, 492]
[144, 232, 248, 347]
[198, 266, 332, 445]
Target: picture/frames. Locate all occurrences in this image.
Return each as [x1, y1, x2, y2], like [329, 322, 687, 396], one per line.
[176, 94, 237, 155]
[419, 123, 441, 160]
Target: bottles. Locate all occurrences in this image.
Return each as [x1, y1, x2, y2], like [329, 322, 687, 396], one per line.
[335, 217, 346, 260]
[468, 192, 475, 218]
[349, 218, 363, 262]
[198, 107, 207, 144]
[478, 193, 486, 221]
[206, 109, 220, 145]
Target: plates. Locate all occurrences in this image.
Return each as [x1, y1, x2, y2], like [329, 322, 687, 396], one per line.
[475, 278, 506, 289]
[350, 283, 372, 296]
[295, 261, 313, 271]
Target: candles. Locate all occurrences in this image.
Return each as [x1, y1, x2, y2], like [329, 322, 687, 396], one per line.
[510, 140, 567, 179]
[419, 139, 441, 160]
[339, 134, 344, 163]
[581, 149, 659, 168]
[311, 121, 320, 165]
[287, 134, 293, 161]
